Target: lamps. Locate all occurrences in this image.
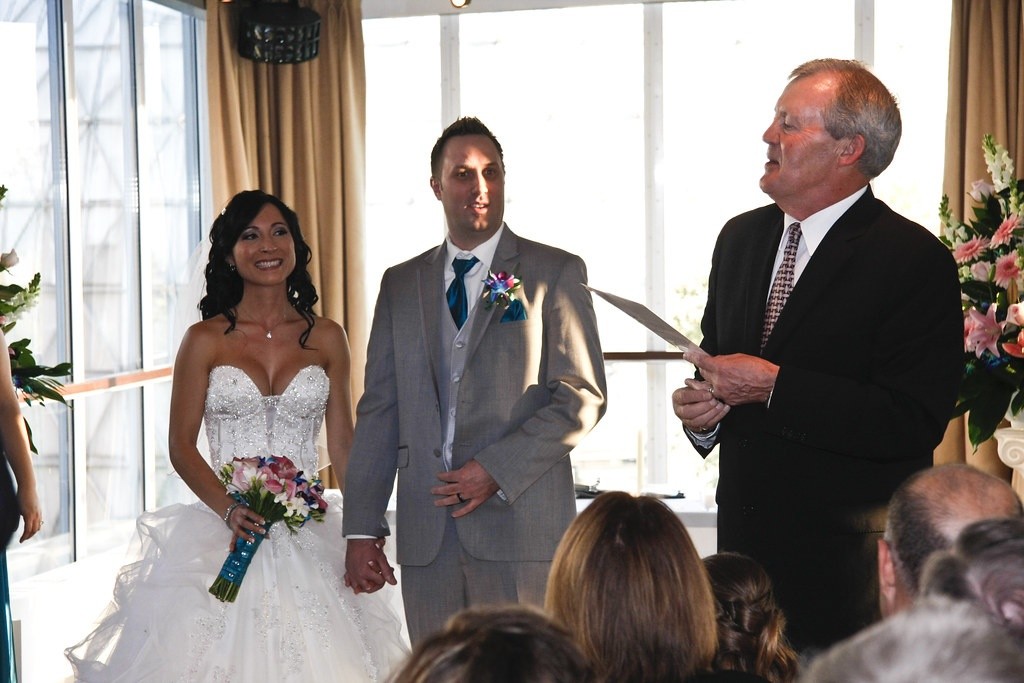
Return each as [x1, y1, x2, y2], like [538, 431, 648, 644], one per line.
[238, 0, 320, 64]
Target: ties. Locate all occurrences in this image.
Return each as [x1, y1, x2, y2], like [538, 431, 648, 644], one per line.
[758, 221, 802, 358]
[445, 255, 480, 331]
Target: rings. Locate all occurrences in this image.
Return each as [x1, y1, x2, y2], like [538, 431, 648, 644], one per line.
[40, 521, 44, 525]
[700, 427, 708, 432]
[457, 493, 465, 504]
[709, 385, 712, 393]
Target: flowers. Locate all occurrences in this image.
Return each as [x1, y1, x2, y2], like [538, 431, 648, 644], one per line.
[481, 269, 522, 311]
[0, 184, 75, 456]
[209, 455, 328, 602]
[936, 134, 1024, 453]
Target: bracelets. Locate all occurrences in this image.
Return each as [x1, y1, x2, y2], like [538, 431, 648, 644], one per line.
[224, 502, 242, 527]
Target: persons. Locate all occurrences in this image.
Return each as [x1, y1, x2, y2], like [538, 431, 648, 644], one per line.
[66, 189, 398, 682]
[0, 326, 43, 683]
[343, 116, 606, 649]
[670, 61, 957, 638]
[391, 463, 1024, 683]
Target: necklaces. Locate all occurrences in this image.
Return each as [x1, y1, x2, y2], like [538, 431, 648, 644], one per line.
[238, 301, 293, 339]
